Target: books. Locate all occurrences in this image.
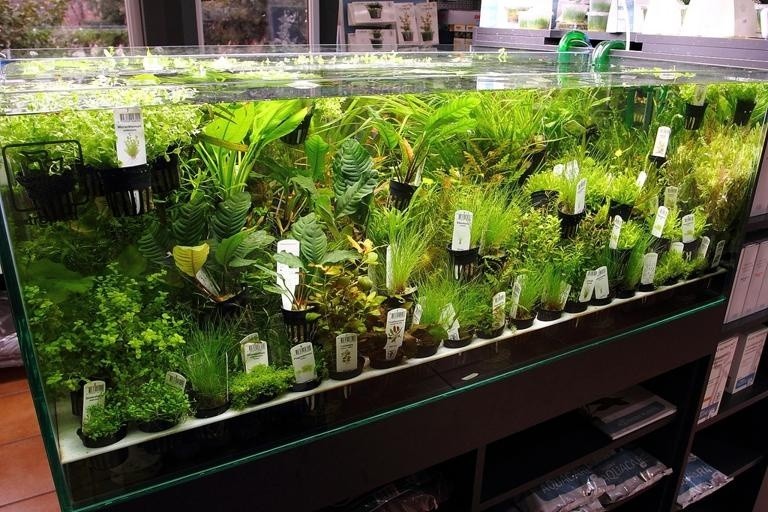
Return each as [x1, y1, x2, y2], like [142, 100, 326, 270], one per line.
[573, 382, 678, 443]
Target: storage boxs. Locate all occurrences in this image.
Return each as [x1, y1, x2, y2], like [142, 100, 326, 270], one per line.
[448, 24, 474, 51]
[0, 45, 768, 511]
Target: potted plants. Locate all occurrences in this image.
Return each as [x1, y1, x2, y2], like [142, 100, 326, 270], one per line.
[399, 10, 415, 41]
[420, 10, 433, 41]
[366, 2, 384, 18]
[369, 28, 383, 47]
[519, 7, 587, 31]
